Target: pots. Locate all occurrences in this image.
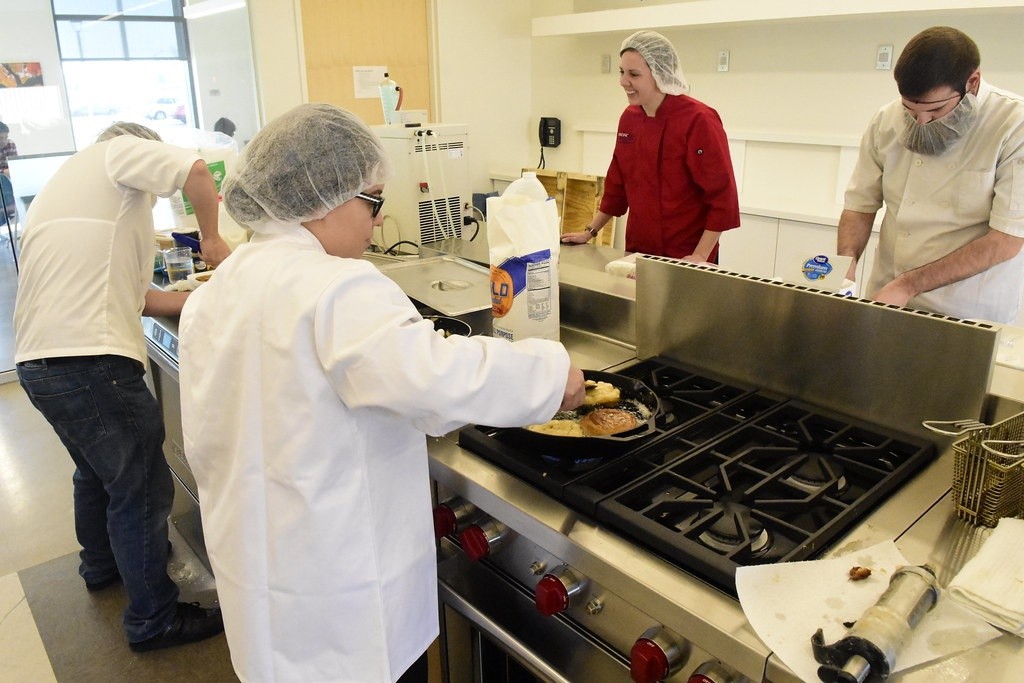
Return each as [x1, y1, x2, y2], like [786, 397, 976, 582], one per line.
[419, 314, 472, 338]
[502, 366, 663, 457]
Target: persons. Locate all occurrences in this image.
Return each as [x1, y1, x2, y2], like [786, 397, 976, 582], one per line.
[0, 122, 17, 179]
[13, 121, 232, 653]
[177, 102, 586, 683]
[560, 30, 740, 266]
[838, 26, 1024, 325]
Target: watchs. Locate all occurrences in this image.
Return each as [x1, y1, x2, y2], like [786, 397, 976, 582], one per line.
[585, 225, 597, 238]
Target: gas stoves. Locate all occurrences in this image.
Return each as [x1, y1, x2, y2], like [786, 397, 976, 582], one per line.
[427, 355, 939, 683]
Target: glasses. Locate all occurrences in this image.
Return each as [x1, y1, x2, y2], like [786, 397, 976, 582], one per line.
[354, 193, 385, 219]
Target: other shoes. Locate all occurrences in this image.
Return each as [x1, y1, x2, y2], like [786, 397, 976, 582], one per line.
[128, 602, 223, 654]
[85, 539, 172, 592]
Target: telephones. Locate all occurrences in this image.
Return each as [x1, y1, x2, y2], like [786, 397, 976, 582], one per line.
[538, 116, 561, 148]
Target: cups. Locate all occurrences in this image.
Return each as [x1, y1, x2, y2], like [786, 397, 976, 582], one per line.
[163, 247, 194, 285]
